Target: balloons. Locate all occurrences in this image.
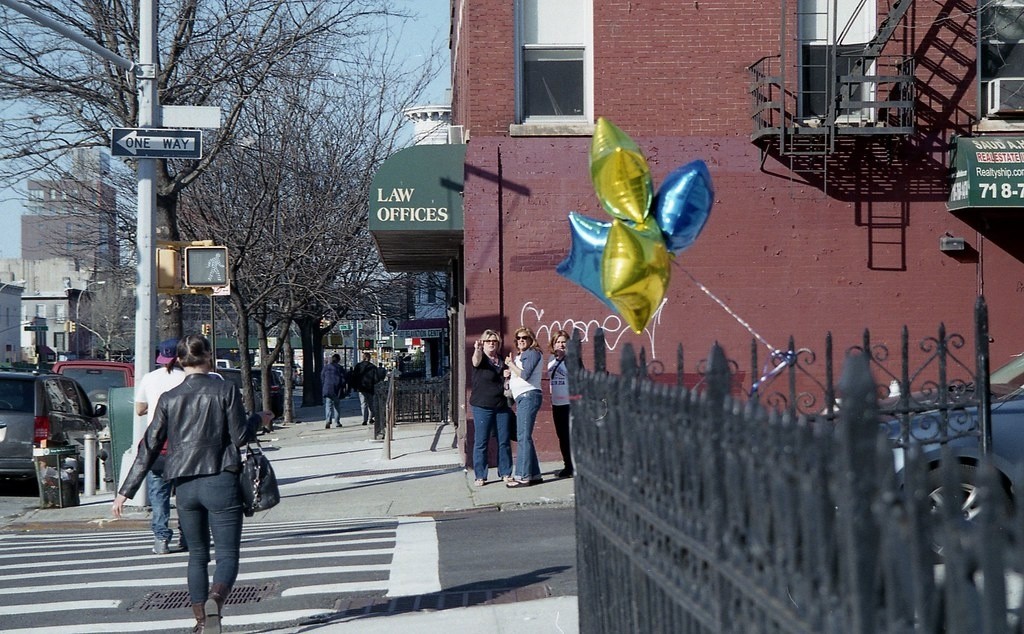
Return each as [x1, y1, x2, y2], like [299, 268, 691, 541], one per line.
[556, 117, 714, 335]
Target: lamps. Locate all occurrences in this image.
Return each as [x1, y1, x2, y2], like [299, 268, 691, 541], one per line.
[450, 296, 459, 314]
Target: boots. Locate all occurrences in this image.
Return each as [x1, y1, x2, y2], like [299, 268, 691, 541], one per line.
[193, 604, 204, 634]
[203, 583, 231, 634]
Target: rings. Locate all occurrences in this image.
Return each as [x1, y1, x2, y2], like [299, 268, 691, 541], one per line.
[112, 505, 115, 508]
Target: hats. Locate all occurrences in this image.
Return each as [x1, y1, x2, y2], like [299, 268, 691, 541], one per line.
[156, 339, 180, 364]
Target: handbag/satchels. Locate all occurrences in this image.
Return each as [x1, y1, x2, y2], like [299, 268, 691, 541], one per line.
[337, 387, 347, 398]
[506, 384, 516, 407]
[492, 409, 518, 443]
[240, 424, 280, 517]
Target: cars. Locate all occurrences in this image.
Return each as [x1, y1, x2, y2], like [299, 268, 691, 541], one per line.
[53, 360, 133, 430]
[0, 367, 104, 484]
[221, 364, 304, 417]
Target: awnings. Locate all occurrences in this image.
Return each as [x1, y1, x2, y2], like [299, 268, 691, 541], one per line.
[206, 337, 303, 349]
[945, 135, 1024, 211]
[369, 144, 464, 273]
[398, 319, 448, 338]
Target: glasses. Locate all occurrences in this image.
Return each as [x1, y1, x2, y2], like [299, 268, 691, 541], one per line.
[515, 336, 531, 340]
[483, 339, 498, 344]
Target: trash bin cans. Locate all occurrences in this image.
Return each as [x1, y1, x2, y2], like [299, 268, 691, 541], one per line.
[31, 444, 80, 509]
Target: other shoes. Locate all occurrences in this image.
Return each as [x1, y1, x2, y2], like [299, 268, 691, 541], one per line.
[325, 421, 332, 429]
[336, 424, 342, 427]
[362, 421, 367, 425]
[559, 464, 573, 477]
[369, 419, 374, 423]
[474, 479, 485, 486]
[502, 475, 515, 482]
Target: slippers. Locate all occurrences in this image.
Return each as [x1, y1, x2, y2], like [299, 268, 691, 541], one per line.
[507, 477, 543, 487]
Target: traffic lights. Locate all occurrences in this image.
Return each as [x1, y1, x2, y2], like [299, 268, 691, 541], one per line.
[205, 323, 210, 336]
[186, 243, 230, 290]
[363, 338, 375, 350]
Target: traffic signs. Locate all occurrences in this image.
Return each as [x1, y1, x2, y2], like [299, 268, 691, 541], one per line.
[109, 126, 203, 160]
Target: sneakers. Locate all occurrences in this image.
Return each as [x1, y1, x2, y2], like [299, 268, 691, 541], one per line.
[177, 536, 187, 550]
[152, 537, 170, 555]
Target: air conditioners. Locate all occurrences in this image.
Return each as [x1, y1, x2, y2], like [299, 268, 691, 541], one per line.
[987, 76, 1024, 115]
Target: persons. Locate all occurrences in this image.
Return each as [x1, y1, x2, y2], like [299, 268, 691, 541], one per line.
[470, 328, 543, 488]
[321, 352, 378, 429]
[376, 363, 388, 384]
[136, 339, 186, 554]
[111, 335, 275, 634]
[547, 330, 585, 478]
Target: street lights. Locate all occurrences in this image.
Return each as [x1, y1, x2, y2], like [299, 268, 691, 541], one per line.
[0, 278, 26, 292]
[76, 280, 106, 362]
[106, 316, 130, 362]
